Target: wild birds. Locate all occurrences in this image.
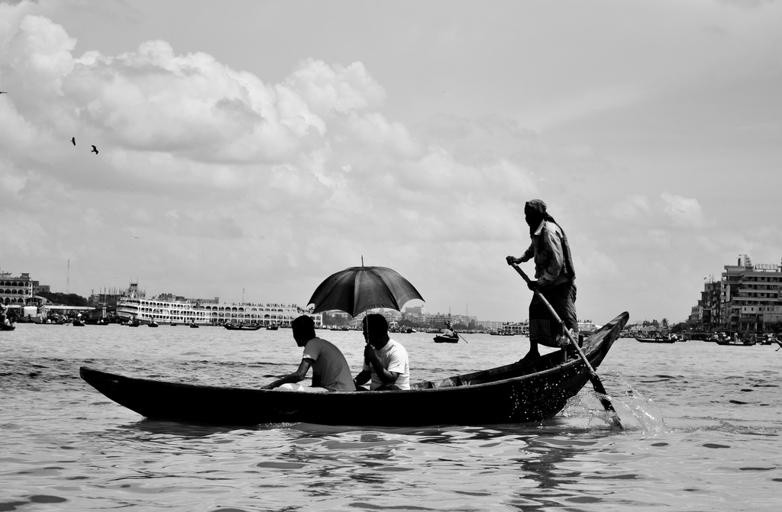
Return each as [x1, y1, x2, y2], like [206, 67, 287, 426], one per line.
[70, 136, 76, 146]
[91, 145, 99, 154]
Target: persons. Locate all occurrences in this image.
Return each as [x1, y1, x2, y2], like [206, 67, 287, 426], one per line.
[444, 321, 453, 331]
[703, 327, 753, 344]
[261, 315, 357, 394]
[506, 199, 580, 364]
[655, 331, 678, 341]
[352, 313, 411, 392]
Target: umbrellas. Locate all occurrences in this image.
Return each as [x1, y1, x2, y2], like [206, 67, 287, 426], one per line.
[306, 255, 425, 343]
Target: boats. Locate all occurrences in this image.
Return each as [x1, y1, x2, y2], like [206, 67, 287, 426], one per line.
[34, 320, 199, 328]
[433, 334, 459, 343]
[78, 311, 630, 427]
[633, 331, 675, 343]
[223, 323, 261, 330]
[714, 334, 782, 348]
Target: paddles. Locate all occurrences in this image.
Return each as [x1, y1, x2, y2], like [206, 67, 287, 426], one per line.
[513, 262, 622, 429]
